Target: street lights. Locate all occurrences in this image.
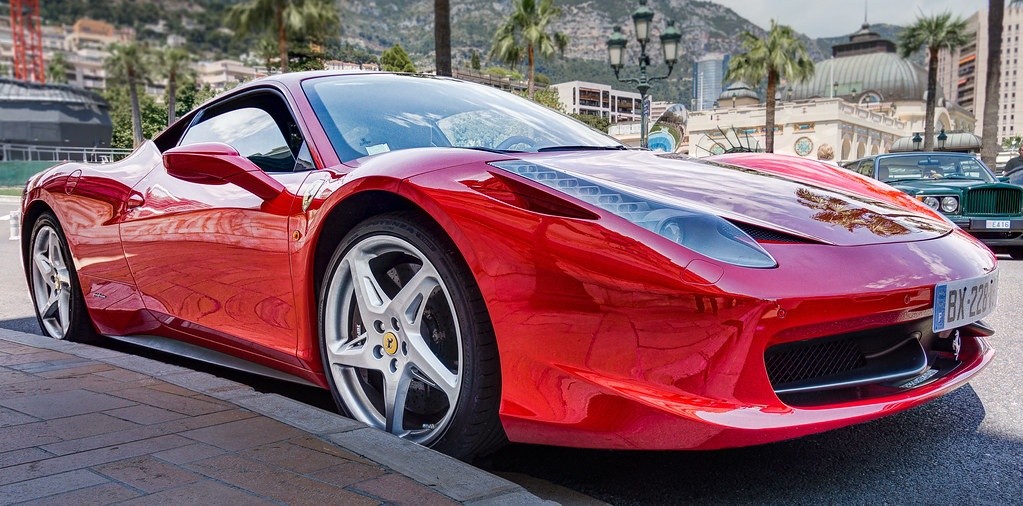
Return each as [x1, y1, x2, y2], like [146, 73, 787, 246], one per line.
[605, 0, 684, 155]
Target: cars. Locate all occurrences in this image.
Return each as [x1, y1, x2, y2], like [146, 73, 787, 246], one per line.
[17, 68, 1007, 458]
[829, 151, 1023, 255]
